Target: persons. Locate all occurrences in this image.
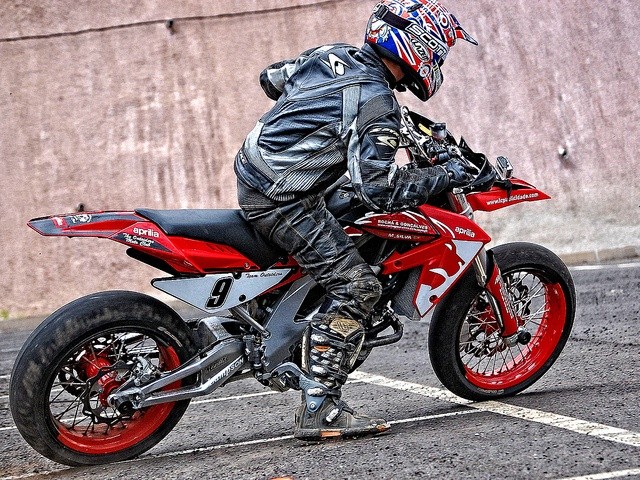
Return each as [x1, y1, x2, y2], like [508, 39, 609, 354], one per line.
[235, 0, 478, 443]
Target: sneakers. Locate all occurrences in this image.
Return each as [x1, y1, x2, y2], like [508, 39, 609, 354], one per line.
[294, 396, 391, 441]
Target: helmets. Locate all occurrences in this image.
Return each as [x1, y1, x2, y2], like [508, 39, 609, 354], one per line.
[364, 0, 478, 102]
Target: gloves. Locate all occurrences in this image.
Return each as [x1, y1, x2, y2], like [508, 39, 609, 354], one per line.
[441, 158, 469, 186]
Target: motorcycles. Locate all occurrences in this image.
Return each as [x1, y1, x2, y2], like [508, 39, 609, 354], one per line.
[9, 107, 576, 466]
